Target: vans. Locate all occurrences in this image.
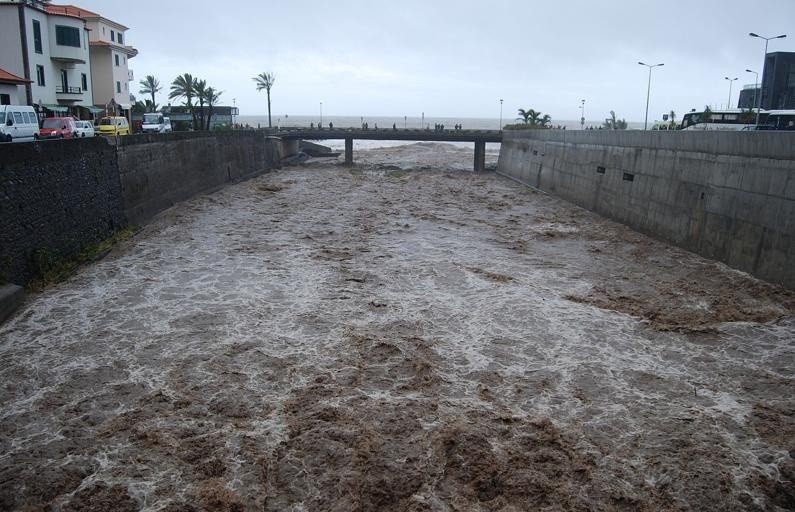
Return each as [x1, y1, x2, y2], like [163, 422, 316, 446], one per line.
[99, 116, 130, 135]
[0, 104, 40, 142]
[141, 113, 172, 133]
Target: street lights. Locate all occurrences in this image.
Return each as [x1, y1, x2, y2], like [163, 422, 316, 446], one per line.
[750, 33, 786, 129]
[726, 77, 738, 105]
[747, 69, 758, 107]
[639, 62, 664, 130]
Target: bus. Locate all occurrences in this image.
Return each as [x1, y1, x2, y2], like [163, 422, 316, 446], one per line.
[681, 107, 764, 131]
[756, 109, 794, 131]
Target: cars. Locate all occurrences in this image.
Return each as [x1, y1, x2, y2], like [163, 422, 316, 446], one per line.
[41, 116, 94, 139]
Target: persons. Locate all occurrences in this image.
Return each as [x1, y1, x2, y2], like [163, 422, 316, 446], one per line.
[318, 122, 321, 127]
[310, 121, 314, 127]
[428, 122, 444, 130]
[650, 117, 676, 130]
[549, 123, 568, 129]
[328, 122, 333, 128]
[375, 123, 377, 130]
[584, 124, 602, 129]
[392, 122, 395, 129]
[361, 122, 368, 130]
[233, 121, 250, 129]
[454, 123, 462, 129]
